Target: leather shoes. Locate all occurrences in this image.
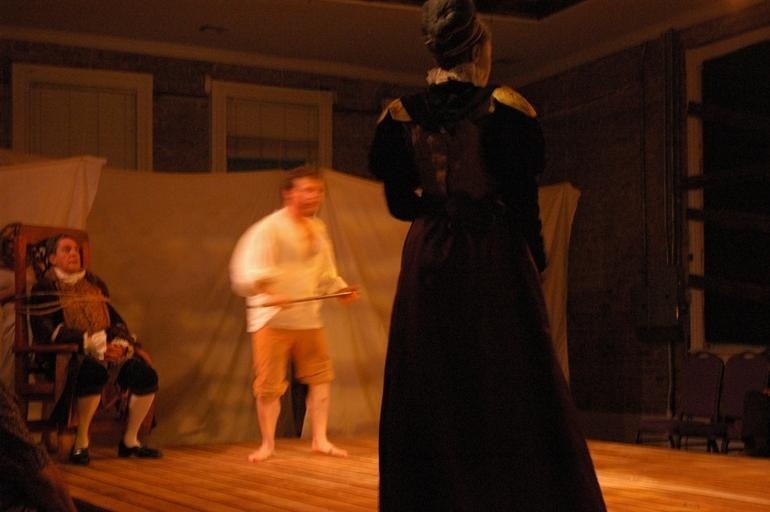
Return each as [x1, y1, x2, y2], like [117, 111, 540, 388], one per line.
[118, 439, 161, 459]
[70, 444, 90, 466]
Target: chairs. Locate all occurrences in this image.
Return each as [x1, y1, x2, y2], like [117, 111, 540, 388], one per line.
[10, 225, 147, 464]
[633, 348, 770, 455]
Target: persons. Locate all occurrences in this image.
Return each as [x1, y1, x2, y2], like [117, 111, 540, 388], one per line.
[365, 1, 611, 510]
[226, 165, 363, 463]
[28, 233, 165, 465]
